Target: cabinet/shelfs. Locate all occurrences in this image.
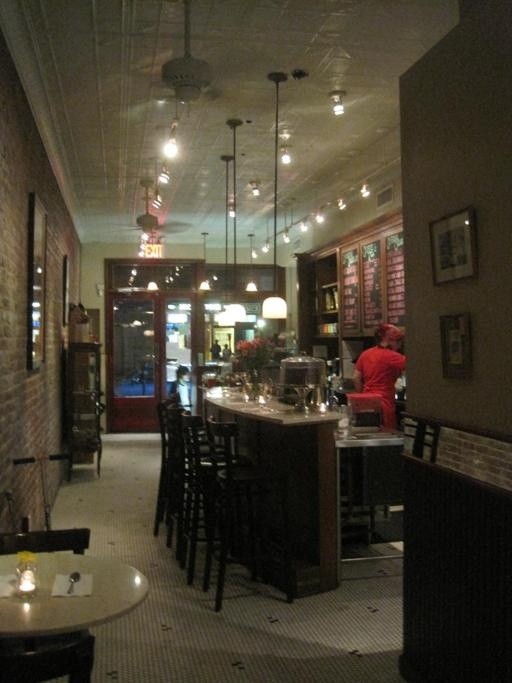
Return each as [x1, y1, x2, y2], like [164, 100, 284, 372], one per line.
[63, 342, 104, 480]
[290, 206, 404, 377]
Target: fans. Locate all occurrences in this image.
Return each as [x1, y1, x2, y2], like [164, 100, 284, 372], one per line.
[108, 179, 192, 234]
[161, 0, 212, 105]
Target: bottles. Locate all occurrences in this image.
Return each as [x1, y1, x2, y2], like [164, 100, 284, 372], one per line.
[14, 549, 37, 600]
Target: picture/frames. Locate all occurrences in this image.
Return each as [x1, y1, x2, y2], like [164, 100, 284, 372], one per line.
[428, 204, 480, 285]
[63, 254, 70, 327]
[438, 311, 474, 379]
[26, 192, 48, 370]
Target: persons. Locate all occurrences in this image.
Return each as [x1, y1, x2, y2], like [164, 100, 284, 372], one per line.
[223, 344, 231, 359]
[172, 363, 191, 412]
[352, 321, 406, 429]
[210, 338, 221, 358]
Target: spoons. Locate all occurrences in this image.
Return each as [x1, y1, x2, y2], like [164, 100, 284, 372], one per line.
[67, 570, 80, 594]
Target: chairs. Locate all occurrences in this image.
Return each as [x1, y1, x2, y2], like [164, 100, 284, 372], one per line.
[0, 528, 95, 683]
[153, 398, 300, 612]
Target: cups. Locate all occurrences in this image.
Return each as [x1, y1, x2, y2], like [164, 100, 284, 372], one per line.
[336, 404, 353, 441]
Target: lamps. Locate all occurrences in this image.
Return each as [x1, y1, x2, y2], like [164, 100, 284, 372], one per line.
[138, 72, 371, 318]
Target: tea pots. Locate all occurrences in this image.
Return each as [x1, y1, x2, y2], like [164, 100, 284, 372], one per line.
[306, 383, 340, 412]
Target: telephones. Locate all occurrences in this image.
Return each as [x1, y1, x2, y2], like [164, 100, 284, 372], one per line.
[340, 223, 407, 338]
